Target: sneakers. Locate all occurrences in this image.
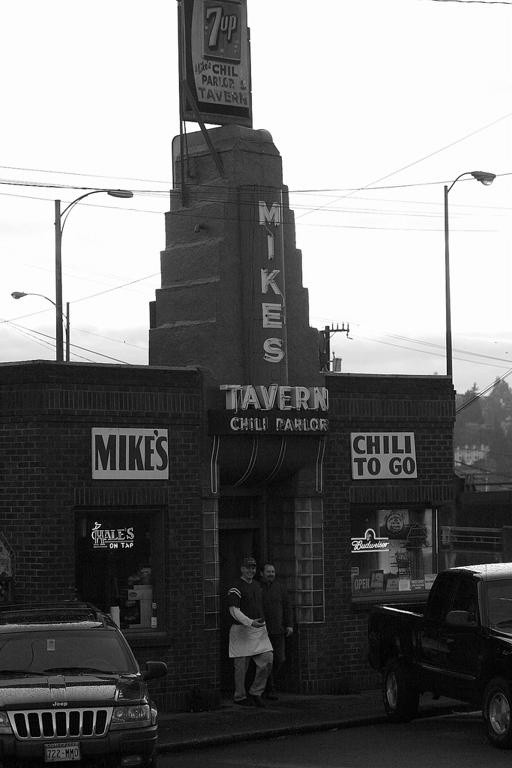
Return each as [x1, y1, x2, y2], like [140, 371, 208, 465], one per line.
[235, 692, 279, 706]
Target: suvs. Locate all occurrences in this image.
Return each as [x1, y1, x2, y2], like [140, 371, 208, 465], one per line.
[0, 597, 173, 767]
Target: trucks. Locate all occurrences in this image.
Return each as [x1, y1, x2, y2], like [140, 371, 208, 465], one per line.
[364, 558, 512, 748]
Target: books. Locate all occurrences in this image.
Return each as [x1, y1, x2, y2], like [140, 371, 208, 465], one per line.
[398, 574, 412, 592]
[369, 568, 385, 595]
[410, 579, 424, 590]
[423, 573, 437, 590]
[383, 576, 399, 593]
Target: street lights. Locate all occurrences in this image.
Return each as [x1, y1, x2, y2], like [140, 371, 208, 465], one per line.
[53, 183, 133, 359]
[440, 167, 499, 379]
[5, 287, 72, 361]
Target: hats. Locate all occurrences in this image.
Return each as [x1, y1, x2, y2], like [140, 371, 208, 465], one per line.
[242, 557, 256, 566]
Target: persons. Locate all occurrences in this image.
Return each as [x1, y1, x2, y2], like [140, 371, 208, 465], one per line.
[257, 559, 296, 702]
[226, 555, 274, 709]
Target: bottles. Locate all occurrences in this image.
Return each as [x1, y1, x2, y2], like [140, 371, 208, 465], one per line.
[150, 602, 158, 630]
